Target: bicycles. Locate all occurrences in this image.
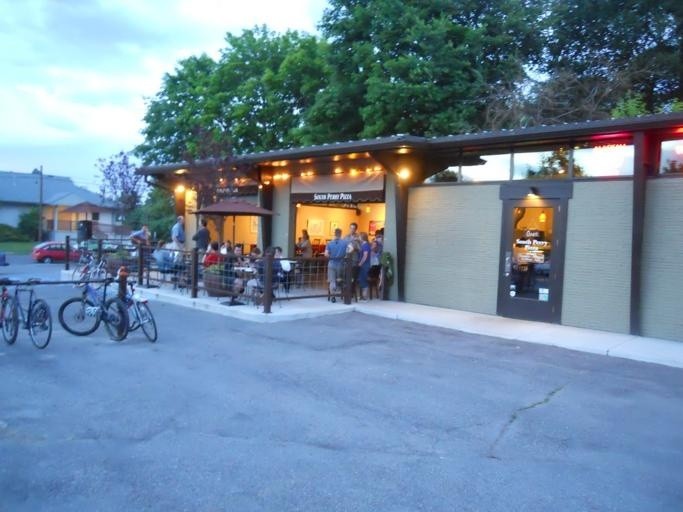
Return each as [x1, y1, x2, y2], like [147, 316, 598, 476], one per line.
[10, 279, 53, 350]
[57, 252, 159, 341]
[0, 276, 20, 345]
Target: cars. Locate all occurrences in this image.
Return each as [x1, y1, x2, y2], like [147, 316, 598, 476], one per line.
[536, 257, 550, 275]
[31, 241, 91, 262]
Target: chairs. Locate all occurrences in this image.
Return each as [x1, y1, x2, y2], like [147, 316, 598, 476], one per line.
[148, 238, 332, 308]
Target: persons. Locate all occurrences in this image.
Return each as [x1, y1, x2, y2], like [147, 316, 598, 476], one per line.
[153, 239, 185, 273]
[195, 217, 384, 303]
[172, 216, 185, 262]
[130, 225, 152, 268]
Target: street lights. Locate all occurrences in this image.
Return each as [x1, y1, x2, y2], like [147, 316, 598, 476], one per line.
[31, 164, 43, 245]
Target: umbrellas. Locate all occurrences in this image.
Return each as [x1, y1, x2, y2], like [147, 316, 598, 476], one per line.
[188, 198, 281, 248]
